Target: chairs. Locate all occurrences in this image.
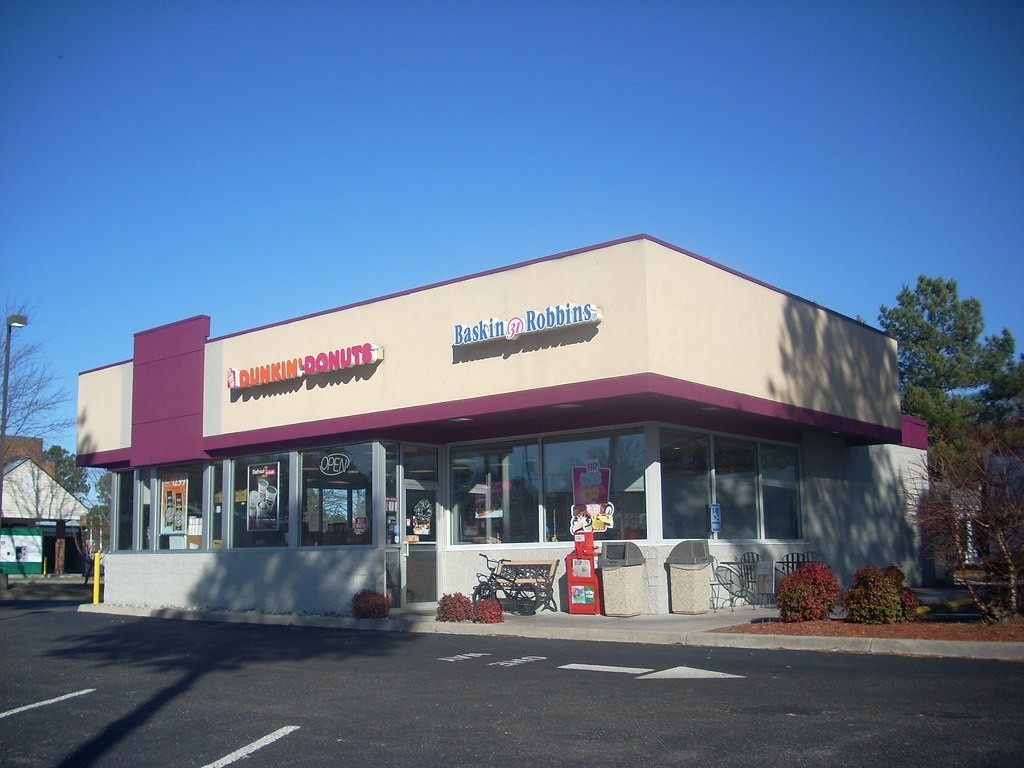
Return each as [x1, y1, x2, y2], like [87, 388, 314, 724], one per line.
[737, 552, 773, 609]
[781, 552, 810, 591]
[709, 555, 735, 613]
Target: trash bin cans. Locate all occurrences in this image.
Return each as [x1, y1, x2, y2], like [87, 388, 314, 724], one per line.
[665, 539, 716, 615]
[598, 541, 647, 618]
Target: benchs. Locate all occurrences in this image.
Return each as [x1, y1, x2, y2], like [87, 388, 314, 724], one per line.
[473, 559, 560, 612]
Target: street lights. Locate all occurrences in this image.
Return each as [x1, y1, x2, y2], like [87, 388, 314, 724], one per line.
[0, 315, 28, 530]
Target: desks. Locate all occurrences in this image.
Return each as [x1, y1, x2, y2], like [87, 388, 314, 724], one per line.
[720, 561, 816, 608]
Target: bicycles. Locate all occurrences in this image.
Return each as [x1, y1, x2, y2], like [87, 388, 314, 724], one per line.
[472, 553, 539, 616]
